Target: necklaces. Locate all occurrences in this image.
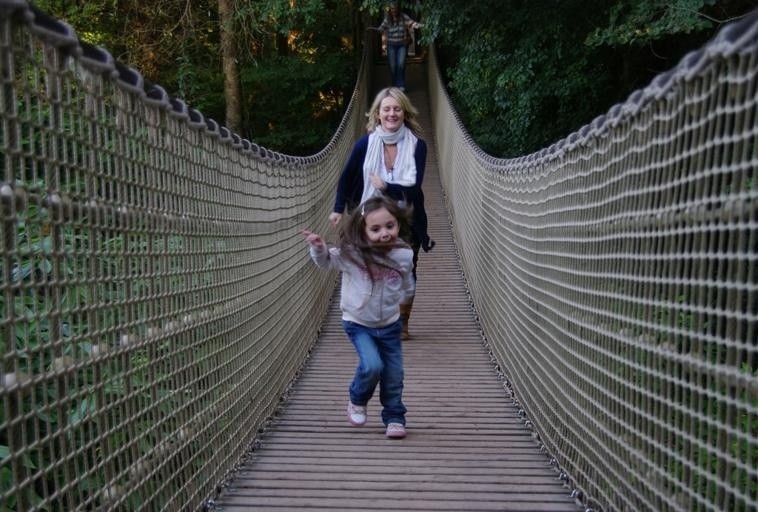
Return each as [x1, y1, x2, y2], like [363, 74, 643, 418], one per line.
[381, 141, 398, 181]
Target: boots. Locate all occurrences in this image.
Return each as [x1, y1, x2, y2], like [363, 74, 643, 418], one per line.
[399, 301, 412, 339]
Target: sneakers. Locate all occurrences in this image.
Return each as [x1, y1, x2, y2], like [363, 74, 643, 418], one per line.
[386, 421, 406, 438]
[347, 397, 367, 425]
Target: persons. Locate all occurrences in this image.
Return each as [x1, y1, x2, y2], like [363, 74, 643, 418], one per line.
[378, 2, 425, 92]
[329, 86, 436, 341]
[301, 196, 415, 439]
